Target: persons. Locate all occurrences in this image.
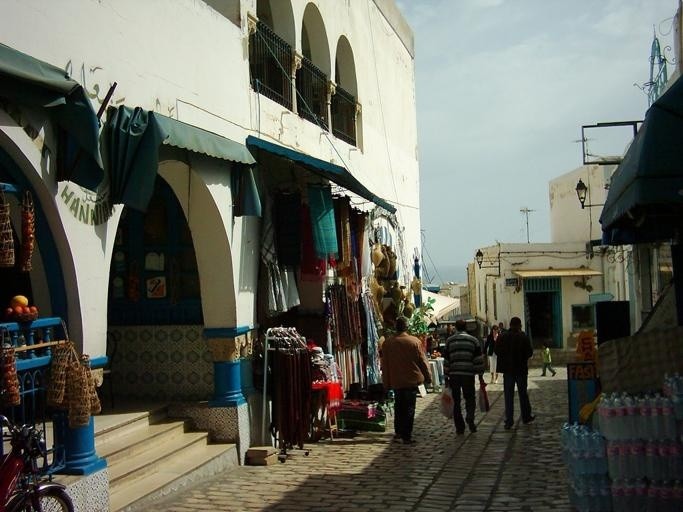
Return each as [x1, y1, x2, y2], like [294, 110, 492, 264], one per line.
[538, 342, 558, 378]
[379, 317, 433, 444]
[444, 317, 534, 435]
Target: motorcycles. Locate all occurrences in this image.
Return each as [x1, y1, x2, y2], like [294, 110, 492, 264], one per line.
[1, 415, 74, 512]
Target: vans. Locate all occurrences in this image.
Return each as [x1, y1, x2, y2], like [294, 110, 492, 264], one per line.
[433, 316, 488, 374]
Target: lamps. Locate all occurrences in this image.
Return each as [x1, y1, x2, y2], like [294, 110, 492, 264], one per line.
[473, 249, 502, 269]
[575, 178, 606, 210]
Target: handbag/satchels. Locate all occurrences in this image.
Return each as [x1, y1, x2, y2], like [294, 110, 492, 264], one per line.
[46, 340, 102, 427]
[478, 378, 489, 412]
[0, 327, 20, 405]
[396, 361, 425, 391]
[439, 388, 454, 418]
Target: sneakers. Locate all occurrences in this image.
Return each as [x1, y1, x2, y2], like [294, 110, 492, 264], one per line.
[470, 425, 476, 432]
[505, 419, 514, 429]
[456, 429, 463, 434]
[393, 435, 415, 444]
[523, 415, 535, 424]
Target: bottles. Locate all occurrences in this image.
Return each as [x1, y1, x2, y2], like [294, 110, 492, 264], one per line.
[559, 372, 682, 511]
